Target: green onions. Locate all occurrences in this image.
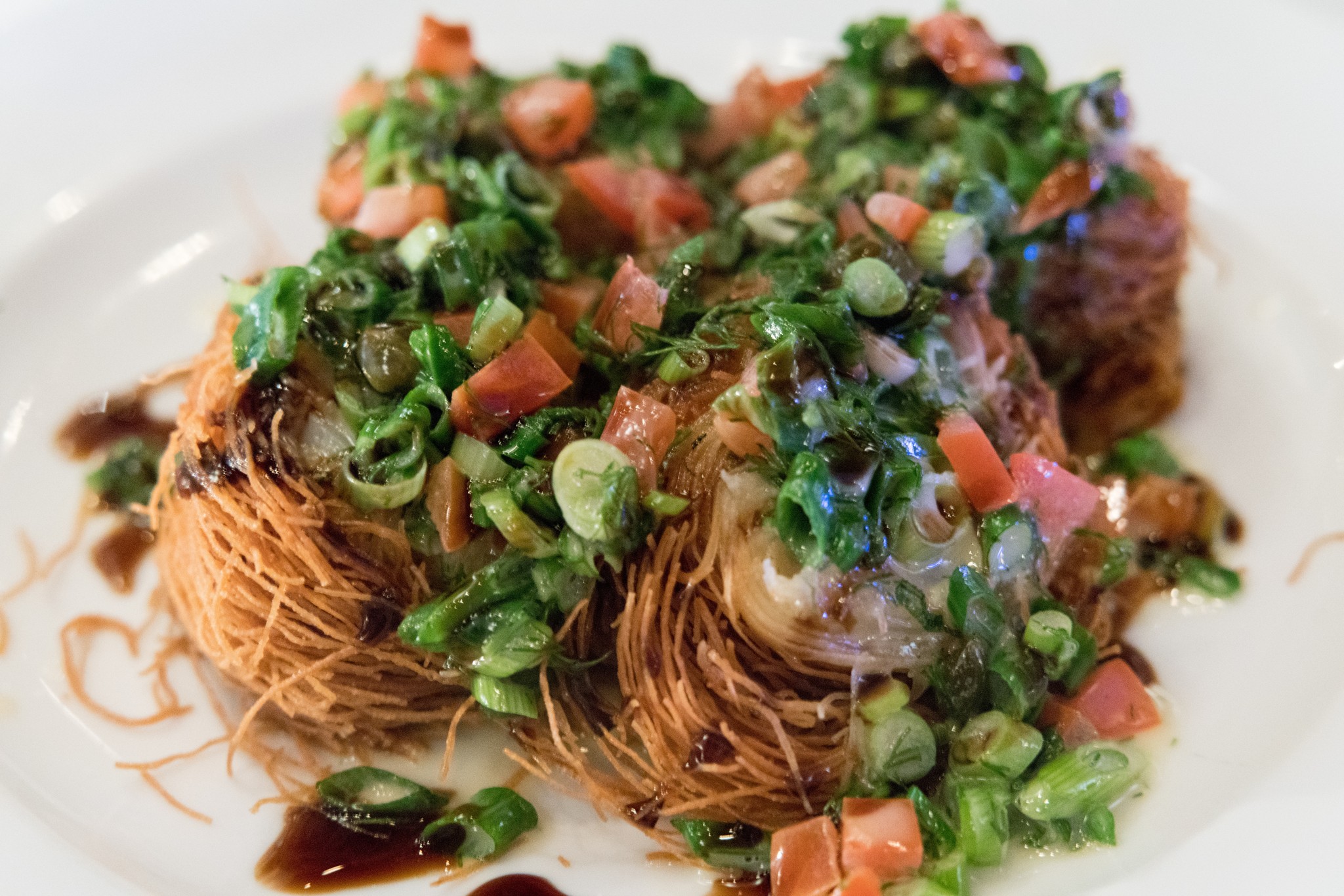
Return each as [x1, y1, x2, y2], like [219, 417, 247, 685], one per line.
[83, 0, 1243, 895]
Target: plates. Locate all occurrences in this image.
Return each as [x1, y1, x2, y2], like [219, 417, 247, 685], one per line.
[0, 0, 1344, 896]
